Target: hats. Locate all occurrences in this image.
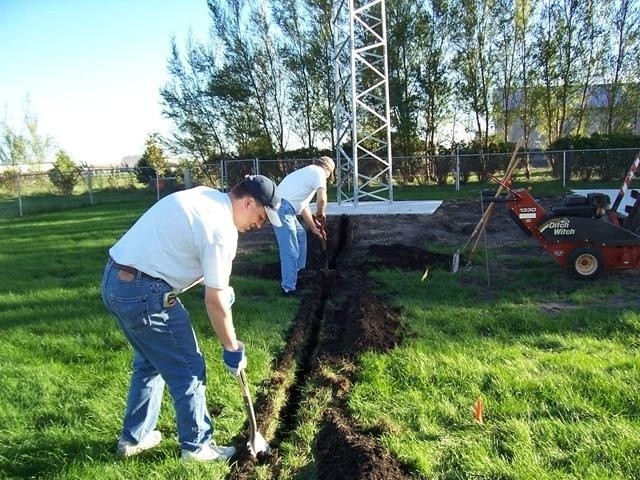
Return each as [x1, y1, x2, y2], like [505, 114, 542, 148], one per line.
[240, 173, 283, 229]
[316, 155, 336, 186]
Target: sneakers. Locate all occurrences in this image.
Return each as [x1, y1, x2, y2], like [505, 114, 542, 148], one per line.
[180, 439, 236, 462]
[117, 430, 162, 457]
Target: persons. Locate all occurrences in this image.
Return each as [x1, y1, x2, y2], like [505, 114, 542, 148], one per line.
[100, 174, 283, 462]
[266, 156, 336, 296]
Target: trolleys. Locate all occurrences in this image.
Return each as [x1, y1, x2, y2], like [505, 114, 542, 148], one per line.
[482, 151, 639, 282]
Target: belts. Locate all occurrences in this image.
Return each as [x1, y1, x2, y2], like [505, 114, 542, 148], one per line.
[106, 255, 138, 277]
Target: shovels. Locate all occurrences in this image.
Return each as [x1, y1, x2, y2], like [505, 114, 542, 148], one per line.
[453, 159, 522, 272]
[318, 218, 337, 281]
[239, 369, 273, 459]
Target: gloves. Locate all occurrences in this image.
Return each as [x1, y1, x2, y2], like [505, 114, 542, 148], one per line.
[223, 339, 247, 376]
[228, 286, 236, 309]
